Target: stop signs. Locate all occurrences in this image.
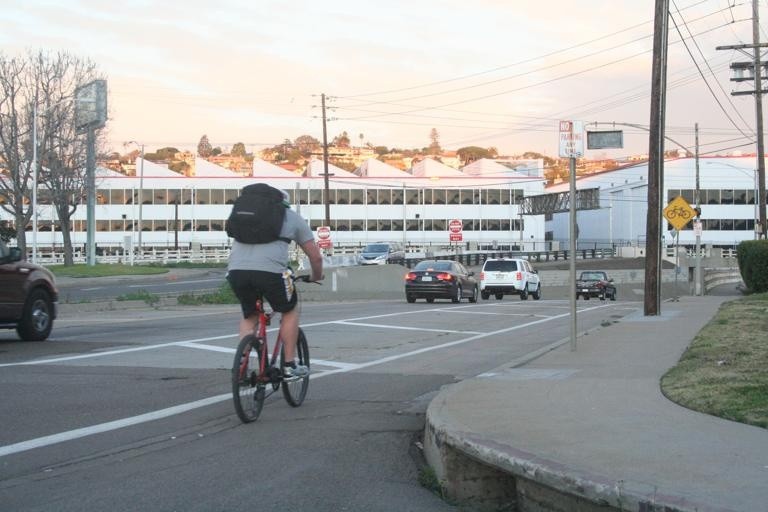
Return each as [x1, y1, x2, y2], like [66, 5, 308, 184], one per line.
[449, 219, 462, 233]
[317, 227, 331, 240]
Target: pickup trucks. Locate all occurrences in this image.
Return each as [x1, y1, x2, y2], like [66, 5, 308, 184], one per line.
[576, 271, 617, 302]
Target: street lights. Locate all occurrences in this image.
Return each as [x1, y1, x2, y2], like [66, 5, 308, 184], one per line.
[31, 98, 96, 268]
[706, 161, 757, 240]
[129, 140, 145, 258]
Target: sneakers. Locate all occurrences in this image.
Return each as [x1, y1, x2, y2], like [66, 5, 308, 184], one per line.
[230, 359, 249, 380]
[282, 363, 309, 382]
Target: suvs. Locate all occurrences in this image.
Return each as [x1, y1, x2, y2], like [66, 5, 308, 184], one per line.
[479, 256, 541, 301]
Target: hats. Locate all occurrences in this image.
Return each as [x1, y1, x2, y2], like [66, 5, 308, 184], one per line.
[280, 189, 290, 203]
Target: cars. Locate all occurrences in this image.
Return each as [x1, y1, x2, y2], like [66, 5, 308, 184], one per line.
[358, 242, 394, 266]
[405, 259, 479, 303]
[387, 248, 407, 266]
[0, 235, 59, 343]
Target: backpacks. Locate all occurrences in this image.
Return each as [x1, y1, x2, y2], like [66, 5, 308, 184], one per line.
[225, 182, 291, 244]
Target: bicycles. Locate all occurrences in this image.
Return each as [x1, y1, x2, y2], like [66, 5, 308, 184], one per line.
[231, 270, 326, 425]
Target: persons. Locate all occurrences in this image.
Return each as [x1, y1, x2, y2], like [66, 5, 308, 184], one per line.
[224, 189, 322, 383]
